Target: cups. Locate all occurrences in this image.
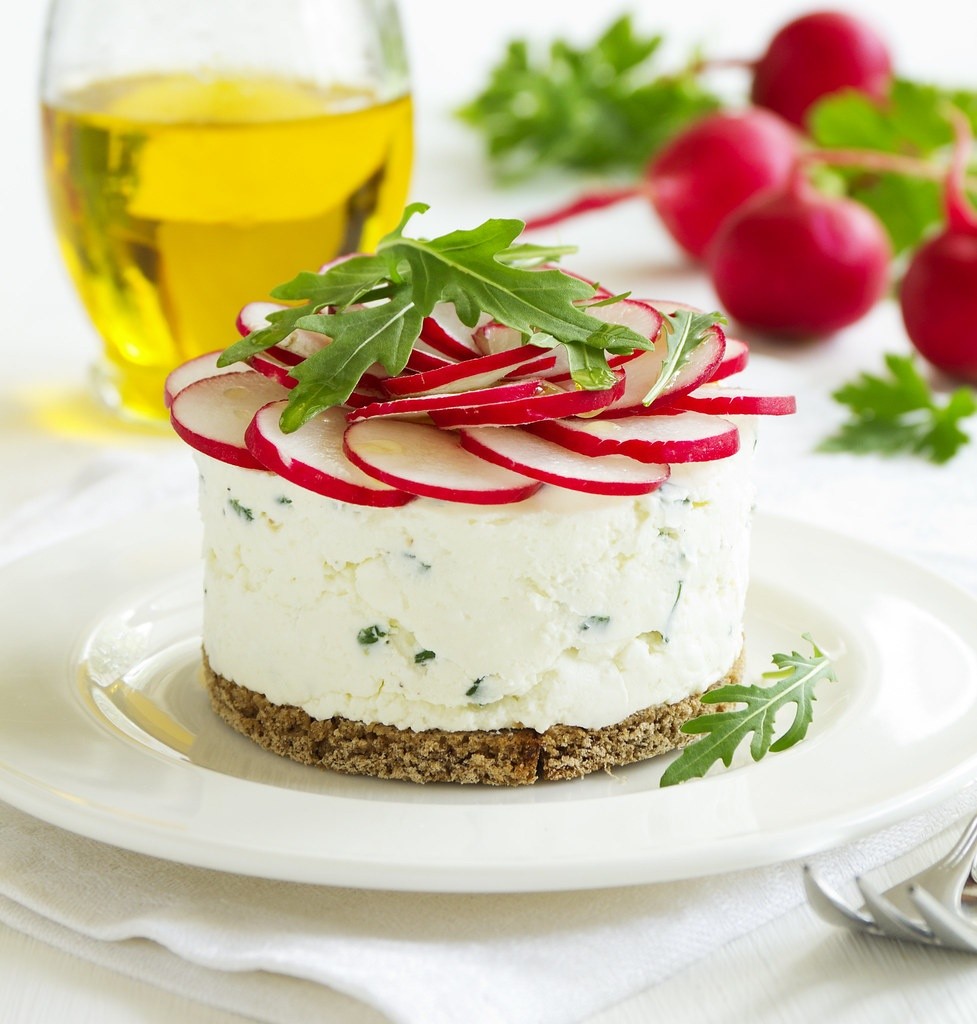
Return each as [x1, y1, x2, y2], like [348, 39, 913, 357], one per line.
[38, 3, 418, 428]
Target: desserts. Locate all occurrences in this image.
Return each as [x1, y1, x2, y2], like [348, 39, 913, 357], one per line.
[159, 205, 798, 783]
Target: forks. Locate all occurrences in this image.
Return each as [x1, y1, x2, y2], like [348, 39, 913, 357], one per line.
[803, 816, 977, 951]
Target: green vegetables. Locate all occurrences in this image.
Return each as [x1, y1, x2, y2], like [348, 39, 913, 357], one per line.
[659, 631, 838, 787]
[446, 7, 977, 471]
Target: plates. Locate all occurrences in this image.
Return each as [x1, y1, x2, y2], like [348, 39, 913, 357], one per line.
[0, 501, 977, 894]
